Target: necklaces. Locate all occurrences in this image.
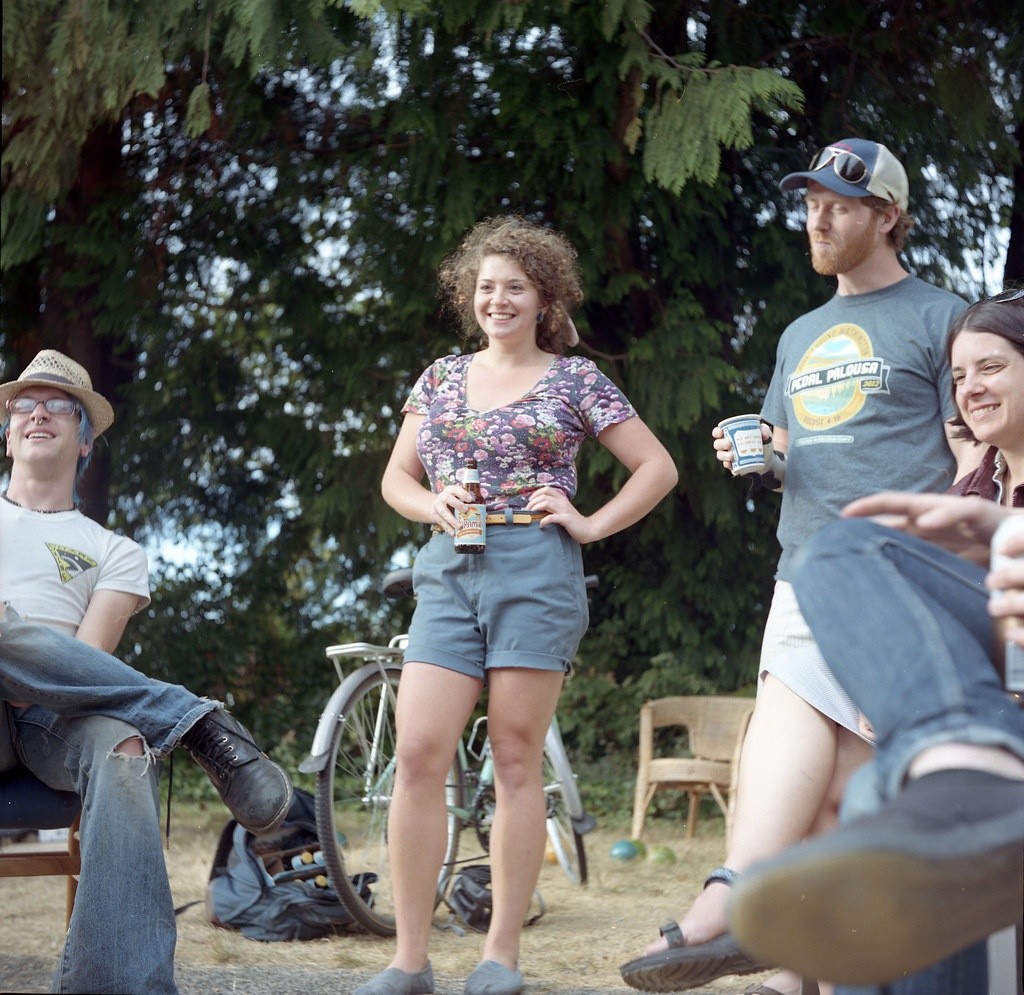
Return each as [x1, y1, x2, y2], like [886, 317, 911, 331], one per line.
[1, 490, 77, 513]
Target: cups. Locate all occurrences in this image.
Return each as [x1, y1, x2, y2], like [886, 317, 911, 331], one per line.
[718, 414, 766, 475]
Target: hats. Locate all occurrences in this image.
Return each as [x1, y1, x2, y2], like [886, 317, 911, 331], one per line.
[779, 138, 909, 213]
[0, 349, 114, 439]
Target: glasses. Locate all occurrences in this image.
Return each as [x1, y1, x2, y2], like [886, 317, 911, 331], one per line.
[6, 397, 81, 415]
[966, 288, 1024, 303]
[808, 147, 895, 204]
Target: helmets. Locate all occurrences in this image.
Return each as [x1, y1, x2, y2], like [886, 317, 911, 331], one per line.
[450, 866, 545, 929]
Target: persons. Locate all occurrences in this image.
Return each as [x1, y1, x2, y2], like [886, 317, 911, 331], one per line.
[0, 349, 293, 995]
[622, 140, 1024, 995]
[353, 212, 679, 995]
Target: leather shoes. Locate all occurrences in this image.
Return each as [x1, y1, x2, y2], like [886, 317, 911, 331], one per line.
[166, 708, 294, 850]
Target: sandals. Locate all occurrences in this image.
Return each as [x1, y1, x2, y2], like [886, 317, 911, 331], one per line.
[742, 974, 819, 995]
[620, 868, 781, 993]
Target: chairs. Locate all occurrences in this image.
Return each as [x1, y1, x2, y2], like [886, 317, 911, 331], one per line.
[630, 694, 754, 844]
[1, 770, 87, 949]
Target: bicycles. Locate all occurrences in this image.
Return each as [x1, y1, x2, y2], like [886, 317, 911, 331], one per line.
[297, 563, 596, 935]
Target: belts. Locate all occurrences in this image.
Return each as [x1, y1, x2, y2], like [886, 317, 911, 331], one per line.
[431, 511, 554, 532]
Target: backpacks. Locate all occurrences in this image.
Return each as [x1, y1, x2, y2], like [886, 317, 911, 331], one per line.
[206, 786, 379, 942]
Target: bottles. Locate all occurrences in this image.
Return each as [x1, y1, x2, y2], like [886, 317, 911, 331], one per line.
[992, 484, 1024, 703]
[454, 457, 485, 554]
[283, 852, 332, 888]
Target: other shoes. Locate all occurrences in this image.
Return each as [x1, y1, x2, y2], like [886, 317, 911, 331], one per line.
[726, 778, 1024, 988]
[350, 960, 434, 995]
[464, 960, 522, 995]
